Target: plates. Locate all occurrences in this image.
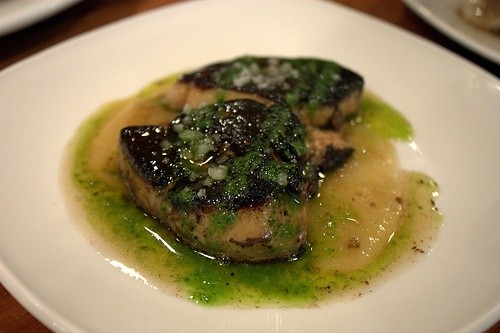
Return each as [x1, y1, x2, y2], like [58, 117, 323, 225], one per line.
[0, 0, 499, 333]
[0, 0, 78, 36]
[405, 0, 500, 65]
